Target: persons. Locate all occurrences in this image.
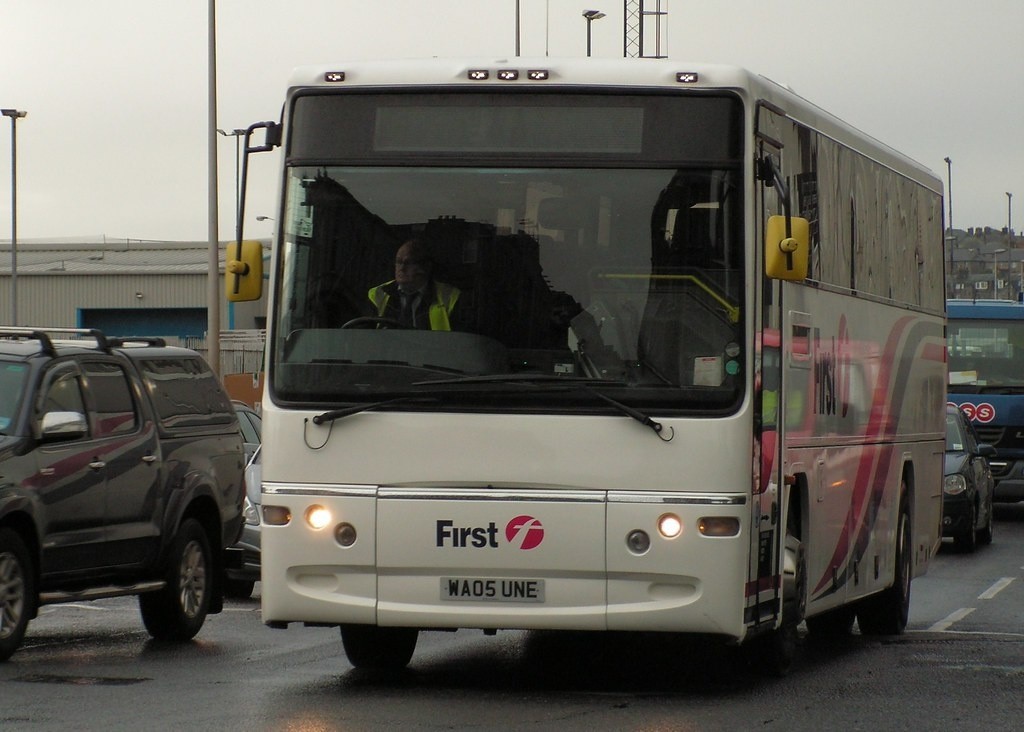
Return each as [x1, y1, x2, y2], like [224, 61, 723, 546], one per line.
[358, 240, 468, 332]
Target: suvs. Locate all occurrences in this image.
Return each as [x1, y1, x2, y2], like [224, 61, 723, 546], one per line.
[230, 398, 262, 464]
[0, 325, 248, 665]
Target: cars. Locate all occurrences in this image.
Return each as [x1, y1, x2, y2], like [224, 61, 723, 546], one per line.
[224, 443, 262, 600]
[942, 402, 996, 553]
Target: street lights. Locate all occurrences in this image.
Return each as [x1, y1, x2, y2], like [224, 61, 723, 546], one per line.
[217, 125, 255, 246]
[1005, 191, 1013, 300]
[944, 157, 955, 299]
[994, 248, 1007, 300]
[582, 9, 607, 56]
[0, 107, 27, 340]
[944, 236, 956, 299]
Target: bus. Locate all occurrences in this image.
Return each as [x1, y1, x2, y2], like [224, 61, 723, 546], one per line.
[946, 297, 1024, 503]
[222, 54, 951, 679]
